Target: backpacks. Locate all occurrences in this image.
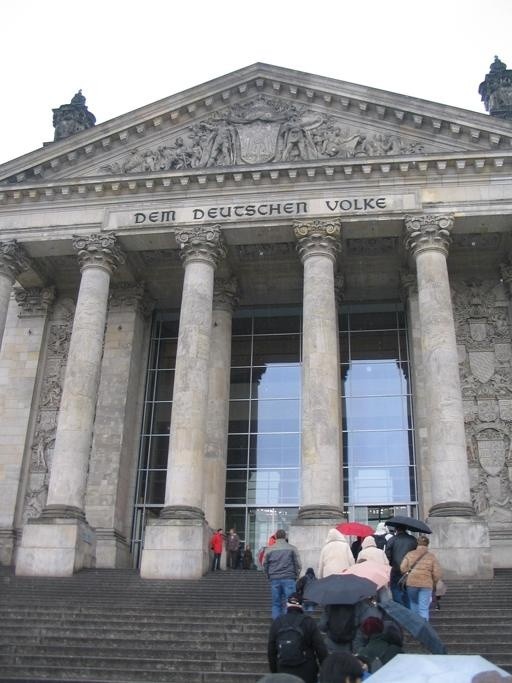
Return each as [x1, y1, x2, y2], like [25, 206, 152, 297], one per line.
[372, 533, 388, 550]
[274, 615, 313, 668]
[364, 644, 393, 674]
[327, 605, 357, 644]
[302, 575, 319, 603]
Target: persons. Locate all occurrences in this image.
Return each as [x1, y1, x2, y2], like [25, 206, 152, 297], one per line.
[226, 527, 240, 569]
[242, 544, 252, 569]
[210, 527, 225, 570]
[254, 514, 447, 681]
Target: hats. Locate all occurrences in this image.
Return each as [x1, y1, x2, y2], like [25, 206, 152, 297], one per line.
[360, 616, 384, 634]
[288, 593, 304, 605]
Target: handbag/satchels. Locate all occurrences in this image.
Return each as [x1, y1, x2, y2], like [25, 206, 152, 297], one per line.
[398, 572, 407, 592]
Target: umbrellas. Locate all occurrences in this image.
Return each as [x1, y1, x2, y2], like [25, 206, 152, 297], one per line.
[360, 648, 512, 683]
